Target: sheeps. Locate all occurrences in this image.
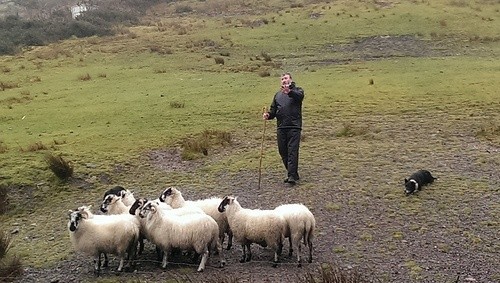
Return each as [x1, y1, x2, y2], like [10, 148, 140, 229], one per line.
[129, 197, 205, 217]
[159, 187, 233, 255]
[218, 196, 287, 267]
[104, 186, 136, 207]
[100, 192, 131, 214]
[273, 203, 315, 267]
[75, 205, 137, 266]
[139, 201, 219, 271]
[67, 210, 139, 273]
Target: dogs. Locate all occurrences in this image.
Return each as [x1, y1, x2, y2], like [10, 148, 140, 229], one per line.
[404, 170, 437, 194]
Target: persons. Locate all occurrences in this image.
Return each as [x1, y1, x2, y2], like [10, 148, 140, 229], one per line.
[262, 72, 304, 185]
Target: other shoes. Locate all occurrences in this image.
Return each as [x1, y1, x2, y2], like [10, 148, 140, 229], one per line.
[288, 176, 295, 183]
[284, 176, 289, 183]
